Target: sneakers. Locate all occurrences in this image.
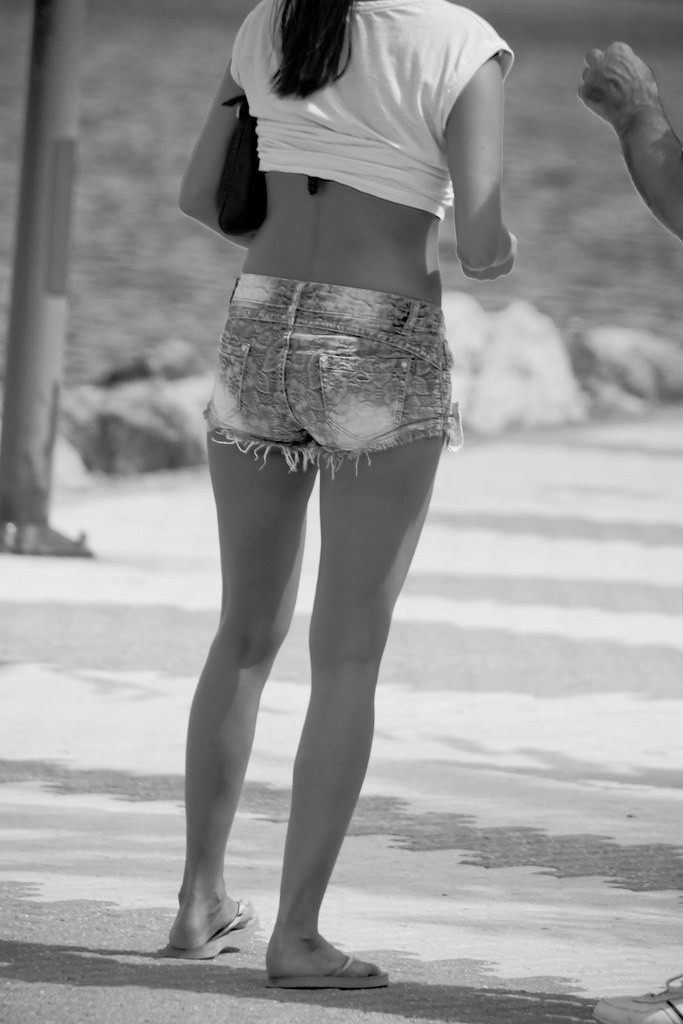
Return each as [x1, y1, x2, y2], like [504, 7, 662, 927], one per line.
[594, 974, 683, 1023]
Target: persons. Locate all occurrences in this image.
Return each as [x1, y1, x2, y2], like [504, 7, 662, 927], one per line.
[160, 0, 516, 987]
[575, 41, 683, 1024]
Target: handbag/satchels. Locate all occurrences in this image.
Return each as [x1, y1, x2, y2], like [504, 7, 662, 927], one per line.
[218, 95, 268, 235]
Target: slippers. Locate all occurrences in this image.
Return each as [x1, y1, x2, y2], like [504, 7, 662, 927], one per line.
[165, 899, 259, 958]
[266, 951, 391, 988]
